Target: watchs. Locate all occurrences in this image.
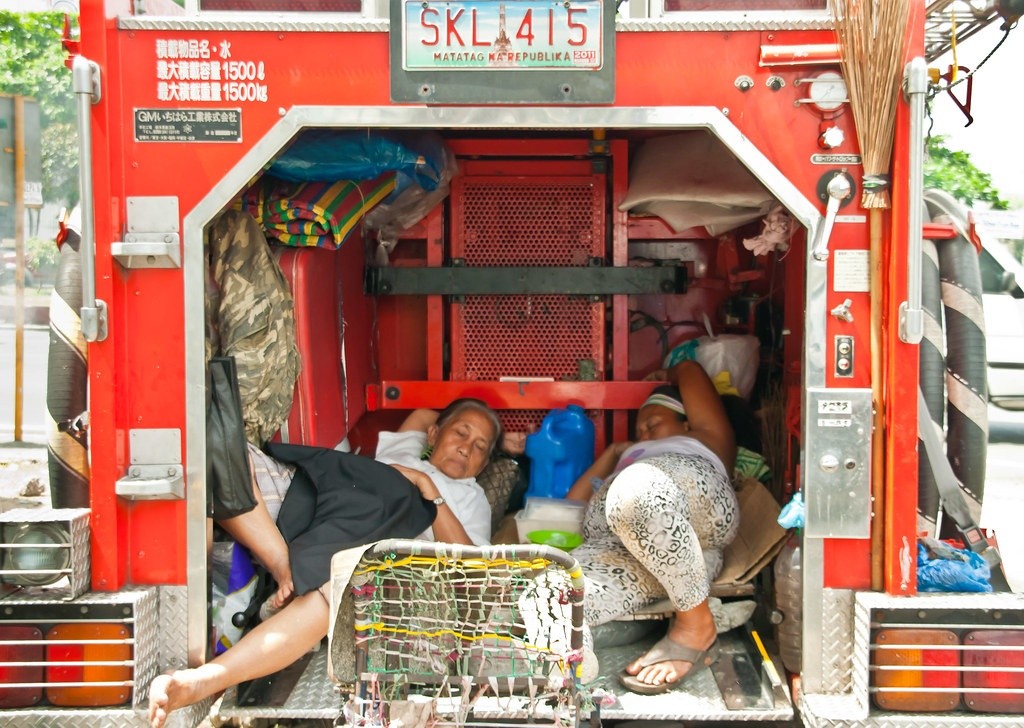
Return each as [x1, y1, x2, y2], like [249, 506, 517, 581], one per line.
[432, 498, 446, 504]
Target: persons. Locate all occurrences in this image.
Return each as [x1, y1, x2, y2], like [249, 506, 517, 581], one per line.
[517, 359, 740, 693]
[148, 397, 533, 728]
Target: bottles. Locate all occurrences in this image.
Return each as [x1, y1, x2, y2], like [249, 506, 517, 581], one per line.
[774, 528, 802, 674]
[524, 398, 594, 505]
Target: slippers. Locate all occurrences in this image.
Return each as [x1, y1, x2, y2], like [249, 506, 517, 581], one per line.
[618, 631, 721, 696]
[471, 636, 528, 693]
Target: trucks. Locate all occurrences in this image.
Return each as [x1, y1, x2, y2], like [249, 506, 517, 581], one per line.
[0, 4, 1024, 728]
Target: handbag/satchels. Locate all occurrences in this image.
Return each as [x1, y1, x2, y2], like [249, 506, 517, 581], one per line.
[204, 355, 258, 522]
